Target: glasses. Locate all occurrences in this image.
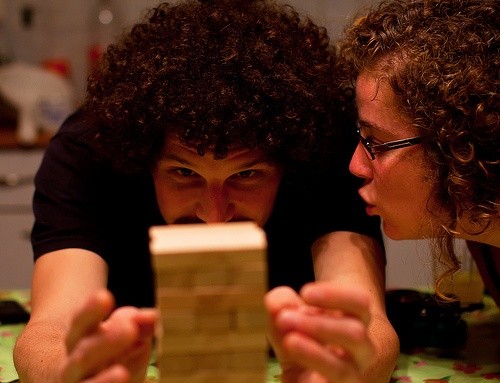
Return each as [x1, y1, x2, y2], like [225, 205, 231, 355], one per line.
[356, 125, 426, 160]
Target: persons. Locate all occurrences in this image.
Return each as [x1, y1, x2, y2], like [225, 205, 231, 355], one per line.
[338, 0, 500, 307]
[13, 0, 401, 383]
